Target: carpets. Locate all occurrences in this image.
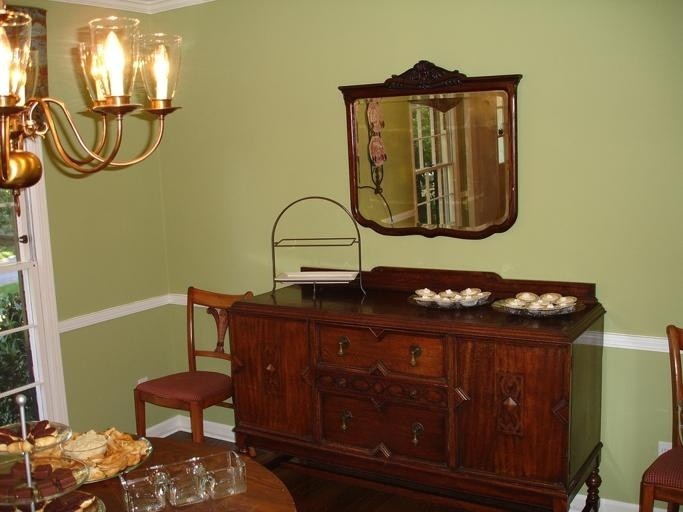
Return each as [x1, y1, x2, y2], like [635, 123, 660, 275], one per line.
[268, 460, 502, 511]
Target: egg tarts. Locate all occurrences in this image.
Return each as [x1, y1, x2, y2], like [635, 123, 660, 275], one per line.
[414, 287, 492, 308]
[504, 291, 577, 309]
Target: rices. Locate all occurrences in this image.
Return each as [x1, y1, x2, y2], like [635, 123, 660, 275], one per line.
[64, 429, 107, 460]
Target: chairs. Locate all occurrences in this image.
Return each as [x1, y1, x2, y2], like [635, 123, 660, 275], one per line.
[638, 326, 682, 512]
[128, 286, 255, 443]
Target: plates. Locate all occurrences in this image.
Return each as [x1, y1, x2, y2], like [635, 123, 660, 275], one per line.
[407, 290, 587, 315]
[276, 270, 359, 284]
[83, 433, 153, 484]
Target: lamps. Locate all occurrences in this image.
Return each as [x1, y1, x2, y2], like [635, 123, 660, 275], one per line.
[0, 0, 184, 218]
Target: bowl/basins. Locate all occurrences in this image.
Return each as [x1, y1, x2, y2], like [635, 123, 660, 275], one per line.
[59, 436, 107, 460]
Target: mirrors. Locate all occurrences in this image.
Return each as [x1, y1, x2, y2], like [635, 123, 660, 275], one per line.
[337, 61, 524, 238]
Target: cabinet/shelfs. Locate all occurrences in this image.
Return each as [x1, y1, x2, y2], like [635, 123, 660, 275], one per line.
[455, 264, 605, 512]
[227, 269, 309, 511]
[312, 265, 453, 510]
